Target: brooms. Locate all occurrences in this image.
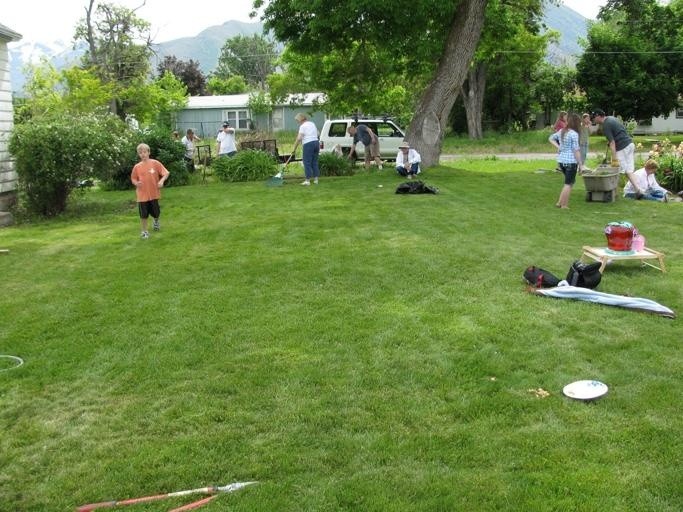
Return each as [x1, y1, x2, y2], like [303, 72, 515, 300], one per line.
[266, 152, 293, 187]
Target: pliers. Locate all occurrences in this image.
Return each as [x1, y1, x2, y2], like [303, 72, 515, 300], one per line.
[75, 481, 257, 512]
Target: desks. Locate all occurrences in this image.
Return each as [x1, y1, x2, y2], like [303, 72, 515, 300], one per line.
[581, 244, 666, 274]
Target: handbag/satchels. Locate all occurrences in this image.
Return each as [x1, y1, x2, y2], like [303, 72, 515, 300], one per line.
[521, 265, 560, 290]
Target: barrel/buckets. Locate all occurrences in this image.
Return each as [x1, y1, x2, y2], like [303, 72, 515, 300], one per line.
[605, 221, 634, 251]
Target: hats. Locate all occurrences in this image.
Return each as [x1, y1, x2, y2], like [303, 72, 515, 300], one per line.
[222, 120, 229, 126]
[398, 141, 410, 149]
[590, 108, 605, 120]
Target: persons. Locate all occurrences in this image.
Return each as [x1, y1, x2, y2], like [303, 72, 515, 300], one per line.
[172, 130, 179, 143]
[546, 113, 583, 210]
[130, 142, 170, 239]
[395, 142, 420, 179]
[621, 159, 673, 202]
[290, 112, 319, 185]
[592, 108, 641, 199]
[578, 112, 591, 174]
[553, 111, 567, 172]
[215, 120, 237, 156]
[346, 124, 382, 171]
[181, 128, 201, 173]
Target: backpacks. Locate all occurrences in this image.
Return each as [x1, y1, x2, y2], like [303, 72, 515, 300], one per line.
[566, 257, 603, 289]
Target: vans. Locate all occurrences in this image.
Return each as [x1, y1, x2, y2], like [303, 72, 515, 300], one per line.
[316, 115, 405, 162]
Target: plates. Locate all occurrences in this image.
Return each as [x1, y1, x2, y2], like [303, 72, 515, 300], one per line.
[563, 378, 608, 401]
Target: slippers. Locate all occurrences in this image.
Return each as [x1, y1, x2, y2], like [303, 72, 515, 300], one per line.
[635, 193, 644, 200]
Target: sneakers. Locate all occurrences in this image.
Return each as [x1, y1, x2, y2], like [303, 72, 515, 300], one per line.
[300, 179, 319, 185]
[663, 193, 669, 204]
[140, 230, 149, 241]
[152, 220, 160, 232]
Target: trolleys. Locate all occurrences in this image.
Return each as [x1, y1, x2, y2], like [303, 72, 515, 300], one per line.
[581, 163, 622, 204]
